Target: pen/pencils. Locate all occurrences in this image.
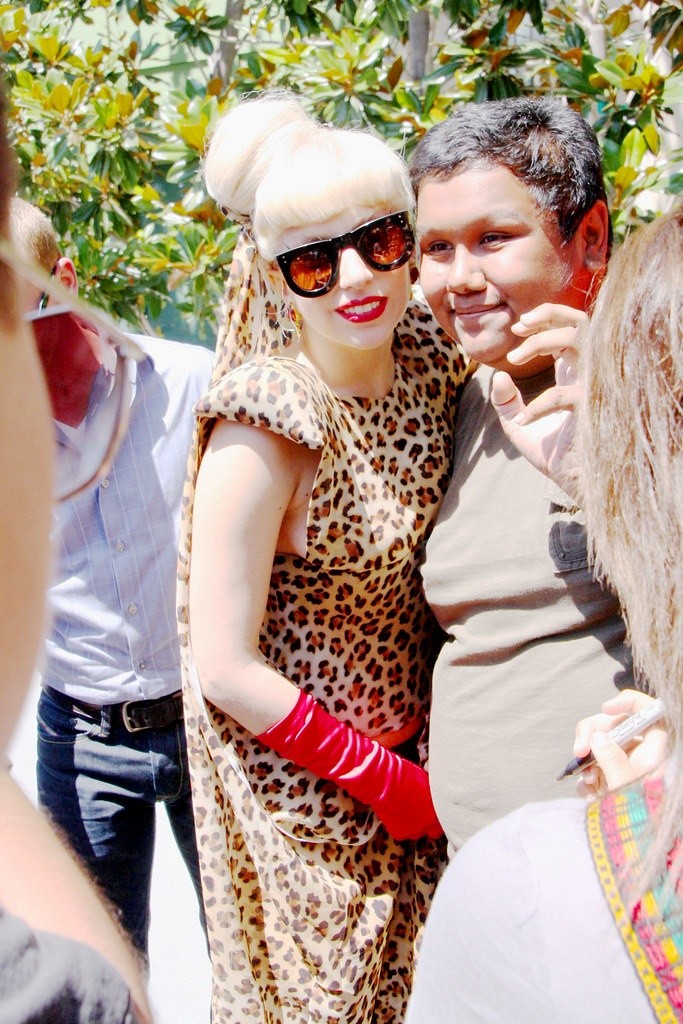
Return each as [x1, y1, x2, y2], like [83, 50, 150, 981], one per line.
[556, 697, 662, 783]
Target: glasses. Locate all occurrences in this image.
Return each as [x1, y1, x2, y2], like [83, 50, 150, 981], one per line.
[0, 244, 150, 504]
[271, 209, 415, 298]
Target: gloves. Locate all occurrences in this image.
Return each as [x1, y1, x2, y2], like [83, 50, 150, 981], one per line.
[255, 688, 444, 841]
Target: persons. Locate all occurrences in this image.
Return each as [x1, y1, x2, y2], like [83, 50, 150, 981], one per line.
[0, 62, 683, 1024]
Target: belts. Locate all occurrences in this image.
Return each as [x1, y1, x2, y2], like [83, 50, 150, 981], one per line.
[44, 684, 184, 733]
